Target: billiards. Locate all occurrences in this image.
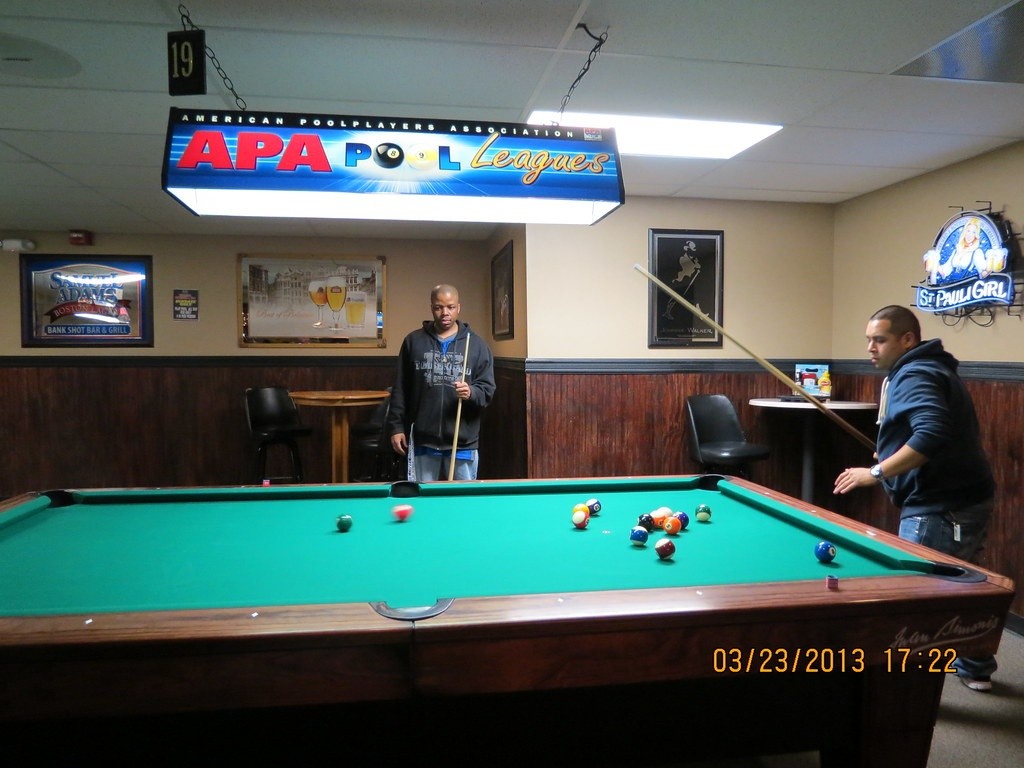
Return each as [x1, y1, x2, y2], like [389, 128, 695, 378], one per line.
[636, 507, 689, 536]
[586, 498, 602, 515]
[629, 525, 649, 546]
[655, 538, 675, 560]
[814, 541, 836, 563]
[394, 505, 409, 520]
[572, 503, 590, 529]
[336, 514, 353, 531]
[695, 503, 711, 521]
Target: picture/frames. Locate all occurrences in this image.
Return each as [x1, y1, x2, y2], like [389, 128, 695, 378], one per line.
[19, 254, 154, 348]
[236, 252, 388, 348]
[647, 228, 724, 348]
[490, 239, 514, 341]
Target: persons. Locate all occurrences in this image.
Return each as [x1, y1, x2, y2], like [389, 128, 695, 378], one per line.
[832, 305, 998, 690]
[386, 283, 497, 482]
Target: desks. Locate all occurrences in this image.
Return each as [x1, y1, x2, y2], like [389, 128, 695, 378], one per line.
[749, 398, 878, 504]
[1, 475, 1019, 768]
[289, 391, 391, 484]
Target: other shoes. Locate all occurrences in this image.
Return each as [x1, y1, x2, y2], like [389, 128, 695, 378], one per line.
[948, 665, 992, 691]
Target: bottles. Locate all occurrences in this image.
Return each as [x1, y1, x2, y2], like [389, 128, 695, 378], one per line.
[793, 372, 816, 395]
[818, 372, 831, 395]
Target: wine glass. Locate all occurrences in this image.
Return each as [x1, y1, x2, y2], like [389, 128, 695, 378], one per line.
[327, 277, 346, 330]
[308, 281, 327, 328]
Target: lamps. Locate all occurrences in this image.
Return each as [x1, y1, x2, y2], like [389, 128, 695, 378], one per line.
[162, 0, 626, 227]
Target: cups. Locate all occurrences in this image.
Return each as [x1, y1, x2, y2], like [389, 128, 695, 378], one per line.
[345, 292, 367, 329]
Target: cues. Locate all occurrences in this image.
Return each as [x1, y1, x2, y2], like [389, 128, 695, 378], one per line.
[633, 262, 878, 453]
[447, 331, 471, 481]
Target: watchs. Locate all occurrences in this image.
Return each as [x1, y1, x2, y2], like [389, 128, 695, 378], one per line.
[870, 463, 885, 482]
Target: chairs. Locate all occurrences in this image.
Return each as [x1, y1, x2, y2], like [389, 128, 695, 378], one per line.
[244, 386, 313, 483]
[683, 394, 772, 483]
[352, 401, 408, 484]
